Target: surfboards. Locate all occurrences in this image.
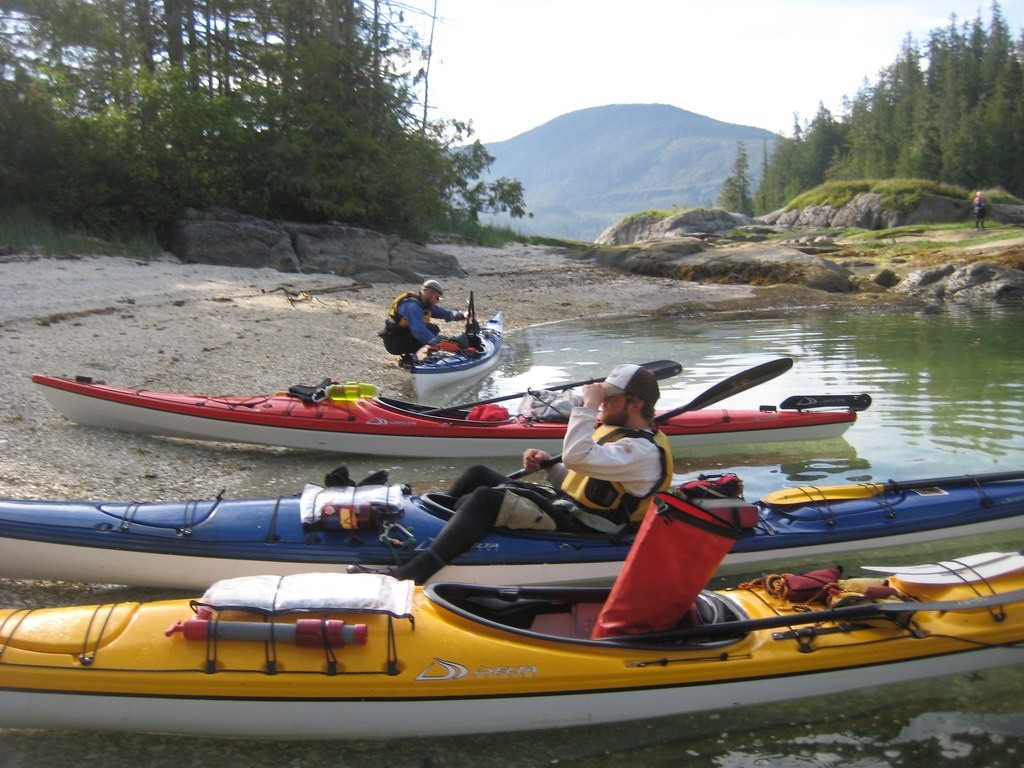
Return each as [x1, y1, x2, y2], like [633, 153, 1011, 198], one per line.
[860, 550, 1024, 574]
[894, 555, 1024, 584]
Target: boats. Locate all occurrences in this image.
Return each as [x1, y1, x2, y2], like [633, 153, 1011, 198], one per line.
[409, 291, 504, 399]
[30, 371, 874, 458]
[0, 467, 1024, 595]
[0, 549, 1024, 742]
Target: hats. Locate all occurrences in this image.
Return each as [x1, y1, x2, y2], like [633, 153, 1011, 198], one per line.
[603, 364, 660, 402]
[422, 280, 445, 298]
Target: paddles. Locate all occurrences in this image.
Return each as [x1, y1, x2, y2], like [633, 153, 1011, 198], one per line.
[594, 587, 1024, 639]
[506, 356, 794, 479]
[466, 290, 481, 348]
[764, 470, 1024, 505]
[418, 361, 682, 414]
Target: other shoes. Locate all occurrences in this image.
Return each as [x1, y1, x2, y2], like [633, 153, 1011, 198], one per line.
[399, 353, 424, 369]
[346, 562, 404, 581]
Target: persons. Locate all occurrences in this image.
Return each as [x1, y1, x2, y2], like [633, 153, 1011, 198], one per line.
[348, 363, 674, 586]
[374, 280, 475, 370]
[973, 191, 988, 231]
